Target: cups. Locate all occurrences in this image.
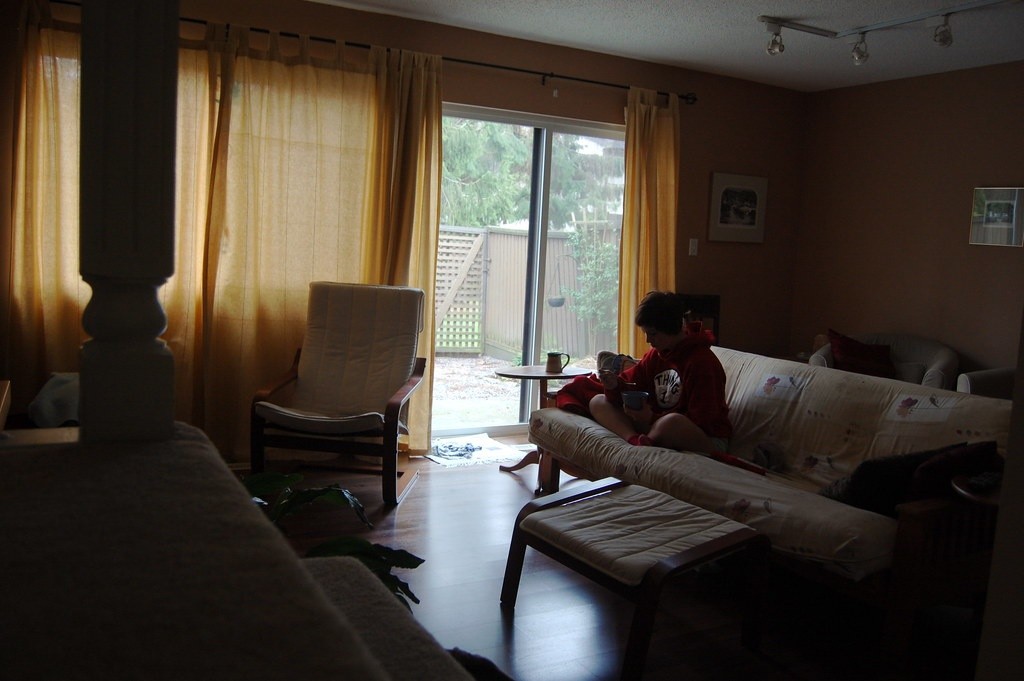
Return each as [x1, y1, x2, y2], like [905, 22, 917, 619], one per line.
[546, 353, 570, 373]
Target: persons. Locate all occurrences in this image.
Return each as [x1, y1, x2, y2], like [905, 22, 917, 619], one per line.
[588, 290, 735, 457]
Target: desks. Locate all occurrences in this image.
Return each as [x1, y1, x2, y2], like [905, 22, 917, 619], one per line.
[494, 363, 592, 411]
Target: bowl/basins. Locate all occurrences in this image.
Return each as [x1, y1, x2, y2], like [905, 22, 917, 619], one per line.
[621, 391, 649, 410]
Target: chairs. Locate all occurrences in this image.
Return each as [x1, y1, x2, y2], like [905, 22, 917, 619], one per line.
[249, 282, 430, 508]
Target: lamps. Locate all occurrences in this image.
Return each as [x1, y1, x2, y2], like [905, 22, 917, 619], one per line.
[756, 12, 953, 67]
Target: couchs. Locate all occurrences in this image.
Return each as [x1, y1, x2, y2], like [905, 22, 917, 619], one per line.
[806, 333, 963, 393]
[526, 343, 1014, 633]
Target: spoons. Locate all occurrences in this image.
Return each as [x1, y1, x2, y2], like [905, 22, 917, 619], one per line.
[604, 369, 636, 385]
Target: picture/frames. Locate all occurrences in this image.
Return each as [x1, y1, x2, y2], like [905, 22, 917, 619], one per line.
[708, 173, 770, 245]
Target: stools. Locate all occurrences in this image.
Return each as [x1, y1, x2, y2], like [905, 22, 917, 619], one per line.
[495, 475, 765, 678]
